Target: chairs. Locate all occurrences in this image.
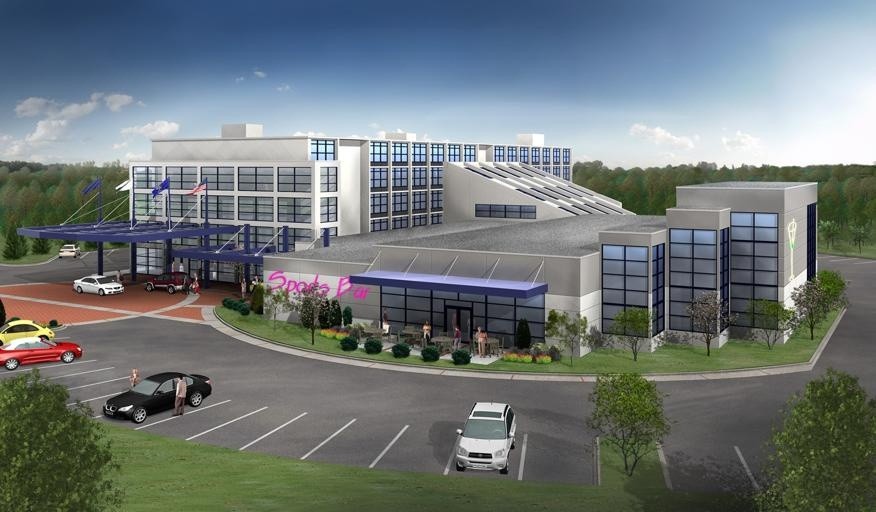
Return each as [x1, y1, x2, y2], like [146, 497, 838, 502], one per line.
[350, 323, 505, 358]
